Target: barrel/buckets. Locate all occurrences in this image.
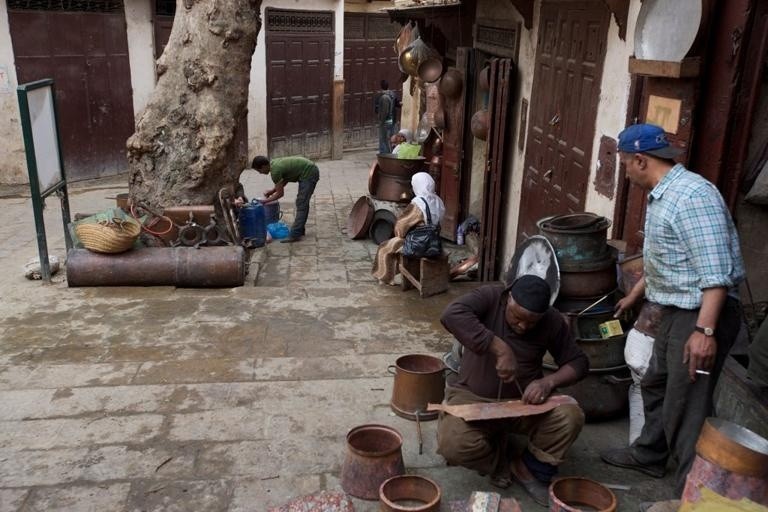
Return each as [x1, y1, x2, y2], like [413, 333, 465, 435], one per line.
[340, 424, 405, 499]
[240, 205, 265, 240]
[548, 476, 617, 512]
[252, 196, 279, 227]
[379, 474, 441, 512]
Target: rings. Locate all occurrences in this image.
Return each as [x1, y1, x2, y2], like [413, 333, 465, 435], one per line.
[541, 397, 544, 400]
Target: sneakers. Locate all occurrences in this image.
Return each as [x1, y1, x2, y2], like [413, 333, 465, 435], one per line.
[633, 477, 681, 501]
[601, 444, 664, 478]
[280, 236, 301, 242]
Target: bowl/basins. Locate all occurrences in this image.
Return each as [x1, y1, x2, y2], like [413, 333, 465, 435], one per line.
[694, 416, 768, 478]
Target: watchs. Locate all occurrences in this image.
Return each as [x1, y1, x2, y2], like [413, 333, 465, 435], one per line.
[695, 326, 716, 337]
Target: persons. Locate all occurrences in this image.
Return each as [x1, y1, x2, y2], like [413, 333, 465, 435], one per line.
[376, 171, 446, 287]
[600, 123, 744, 477]
[437, 273, 583, 505]
[252, 155, 319, 243]
[375, 78, 396, 154]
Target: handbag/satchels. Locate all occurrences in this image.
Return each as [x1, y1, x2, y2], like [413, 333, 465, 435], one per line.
[400, 223, 443, 255]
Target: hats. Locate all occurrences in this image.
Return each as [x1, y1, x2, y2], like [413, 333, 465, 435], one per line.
[618, 125, 680, 161]
[511, 275, 552, 313]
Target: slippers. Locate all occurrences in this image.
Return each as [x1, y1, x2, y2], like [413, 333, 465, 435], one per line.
[490, 451, 549, 506]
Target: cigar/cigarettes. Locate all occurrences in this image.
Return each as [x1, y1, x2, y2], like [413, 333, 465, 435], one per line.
[688, 369, 711, 375]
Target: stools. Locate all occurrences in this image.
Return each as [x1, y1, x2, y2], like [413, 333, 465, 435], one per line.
[397, 249, 449, 299]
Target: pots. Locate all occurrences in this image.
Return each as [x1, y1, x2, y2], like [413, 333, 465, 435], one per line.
[399, 46, 490, 139]
[347, 154, 426, 245]
[536, 213, 635, 426]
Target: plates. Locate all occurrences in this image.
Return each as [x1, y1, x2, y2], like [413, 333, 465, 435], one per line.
[631, 1, 705, 64]
